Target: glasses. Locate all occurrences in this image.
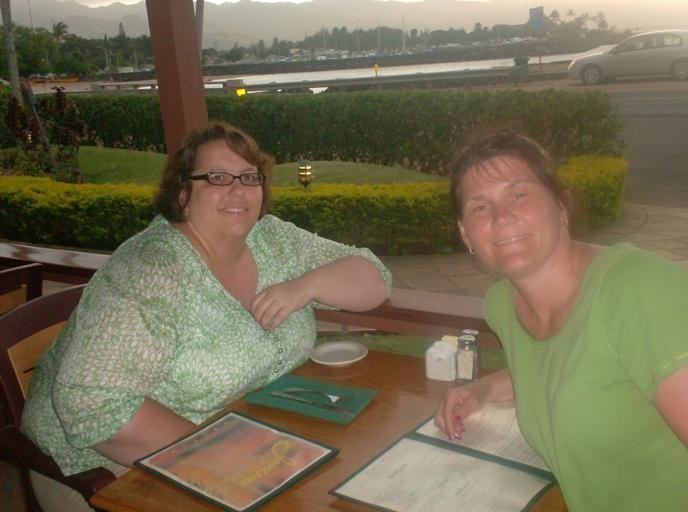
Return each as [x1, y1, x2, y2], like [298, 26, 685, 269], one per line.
[188, 173, 266, 187]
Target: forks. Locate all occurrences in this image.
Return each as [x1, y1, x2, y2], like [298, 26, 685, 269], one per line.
[277, 384, 357, 404]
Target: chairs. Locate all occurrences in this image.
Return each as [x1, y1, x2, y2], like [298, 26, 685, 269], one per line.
[1, 280, 118, 511]
[0, 256, 45, 318]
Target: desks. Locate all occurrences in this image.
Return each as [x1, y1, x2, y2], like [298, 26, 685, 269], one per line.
[88, 342, 571, 512]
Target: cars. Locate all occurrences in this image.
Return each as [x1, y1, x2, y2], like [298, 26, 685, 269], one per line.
[568, 29, 687, 86]
[200, 46, 413, 71]
[28, 62, 156, 84]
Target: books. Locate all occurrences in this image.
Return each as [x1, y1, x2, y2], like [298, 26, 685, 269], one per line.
[328, 393, 557, 512]
[129, 411, 342, 509]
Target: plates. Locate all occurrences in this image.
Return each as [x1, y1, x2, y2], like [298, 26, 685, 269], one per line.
[307, 339, 370, 369]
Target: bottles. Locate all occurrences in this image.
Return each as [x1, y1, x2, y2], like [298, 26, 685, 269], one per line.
[454, 329, 483, 385]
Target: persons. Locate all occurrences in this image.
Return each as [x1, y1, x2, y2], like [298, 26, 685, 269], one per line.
[433, 129, 688, 512]
[19, 119, 391, 512]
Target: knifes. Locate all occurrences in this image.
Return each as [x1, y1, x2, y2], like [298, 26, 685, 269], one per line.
[264, 391, 360, 421]
